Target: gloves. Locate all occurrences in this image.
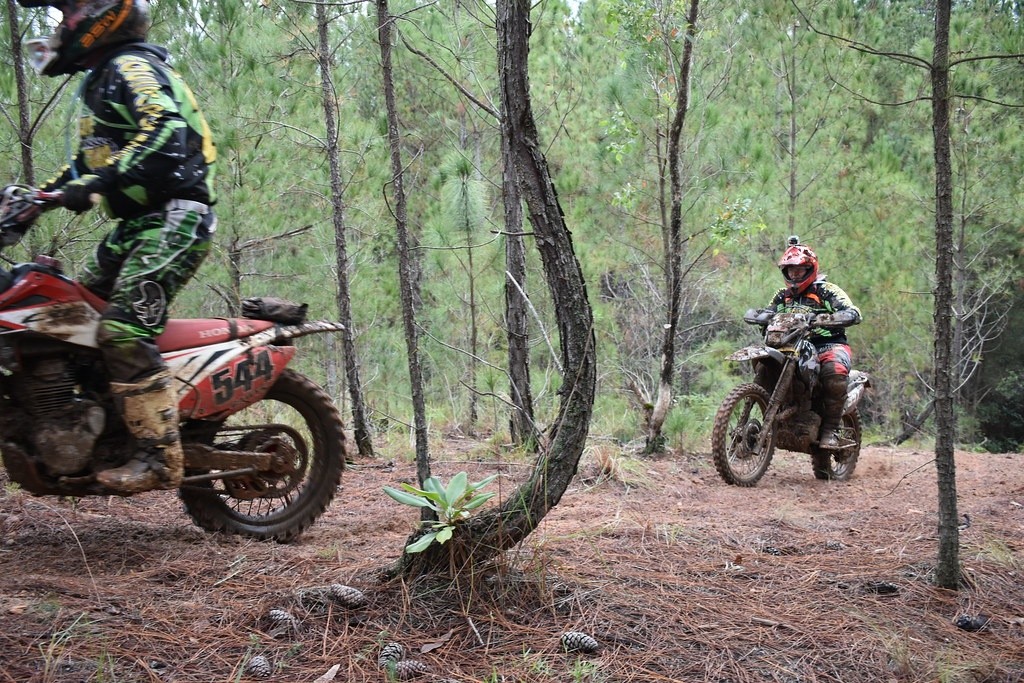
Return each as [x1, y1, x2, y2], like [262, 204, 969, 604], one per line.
[817, 313, 831, 323]
[62, 173, 104, 212]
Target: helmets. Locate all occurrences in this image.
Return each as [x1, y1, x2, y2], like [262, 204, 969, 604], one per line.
[778, 243, 818, 296]
[17, 0, 151, 78]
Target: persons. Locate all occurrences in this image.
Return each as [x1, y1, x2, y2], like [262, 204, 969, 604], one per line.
[759, 235, 862, 450]
[0, 0, 218, 495]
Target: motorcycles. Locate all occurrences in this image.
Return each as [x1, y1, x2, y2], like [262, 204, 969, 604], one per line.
[0, 184, 348, 542]
[710, 307, 871, 487]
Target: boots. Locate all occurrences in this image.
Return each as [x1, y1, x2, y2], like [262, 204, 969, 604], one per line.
[819, 392, 847, 449]
[96, 368, 183, 491]
